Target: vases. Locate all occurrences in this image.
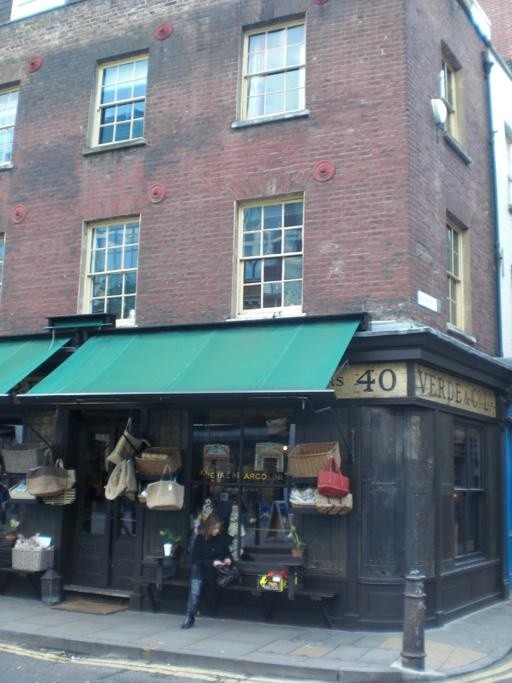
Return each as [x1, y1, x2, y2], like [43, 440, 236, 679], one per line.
[292, 548, 304, 558]
[7, 532, 15, 542]
[164, 539, 174, 559]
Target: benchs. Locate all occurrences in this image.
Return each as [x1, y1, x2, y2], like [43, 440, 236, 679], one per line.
[0, 560, 53, 602]
[134, 574, 340, 630]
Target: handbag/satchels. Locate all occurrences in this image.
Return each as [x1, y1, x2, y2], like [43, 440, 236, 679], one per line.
[147, 481, 185, 512]
[215, 565, 241, 587]
[107, 418, 142, 464]
[317, 471, 353, 496]
[26, 449, 77, 505]
[315, 493, 353, 515]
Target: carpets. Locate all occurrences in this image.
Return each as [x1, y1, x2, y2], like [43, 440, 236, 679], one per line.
[50, 597, 129, 615]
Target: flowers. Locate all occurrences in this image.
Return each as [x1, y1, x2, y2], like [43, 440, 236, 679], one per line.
[0, 516, 23, 533]
[156, 524, 180, 543]
[284, 525, 309, 548]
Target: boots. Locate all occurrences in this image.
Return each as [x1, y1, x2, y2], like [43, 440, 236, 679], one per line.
[182, 580, 202, 629]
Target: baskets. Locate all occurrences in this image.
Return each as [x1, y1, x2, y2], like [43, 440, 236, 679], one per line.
[288, 442, 341, 478]
[290, 487, 316, 508]
[12, 549, 55, 572]
[0, 444, 51, 473]
[135, 446, 182, 475]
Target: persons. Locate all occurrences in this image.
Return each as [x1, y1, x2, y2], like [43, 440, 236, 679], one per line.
[181, 514, 235, 629]
